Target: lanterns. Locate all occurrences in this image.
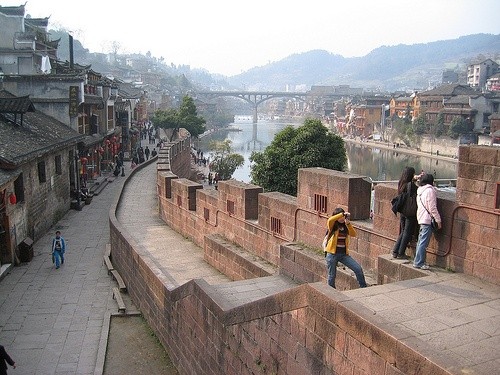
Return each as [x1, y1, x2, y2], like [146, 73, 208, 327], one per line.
[112, 136, 117, 142]
[105, 140, 109, 144]
[9, 194, 16, 206]
[82, 157, 87, 164]
[99, 149, 105, 154]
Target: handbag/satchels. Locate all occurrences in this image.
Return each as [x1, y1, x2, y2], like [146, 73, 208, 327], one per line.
[51, 253, 55, 264]
[396, 193, 407, 213]
[431, 218, 438, 229]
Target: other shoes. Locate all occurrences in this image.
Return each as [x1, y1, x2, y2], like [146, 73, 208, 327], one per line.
[423, 262, 430, 266]
[413, 264, 430, 270]
[393, 253, 397, 258]
[397, 254, 411, 259]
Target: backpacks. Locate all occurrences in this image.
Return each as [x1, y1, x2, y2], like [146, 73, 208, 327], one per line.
[323, 222, 350, 252]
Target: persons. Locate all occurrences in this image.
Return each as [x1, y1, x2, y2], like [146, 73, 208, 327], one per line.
[152, 148, 157, 157]
[138, 147, 144, 162]
[369, 209, 374, 220]
[392, 166, 422, 260]
[145, 146, 150, 160]
[131, 161, 137, 169]
[328, 205, 366, 290]
[116, 158, 122, 169]
[0, 345, 16, 375]
[140, 122, 163, 147]
[193, 148, 219, 186]
[52, 231, 66, 269]
[413, 173, 442, 269]
[120, 155, 124, 166]
[133, 155, 138, 164]
